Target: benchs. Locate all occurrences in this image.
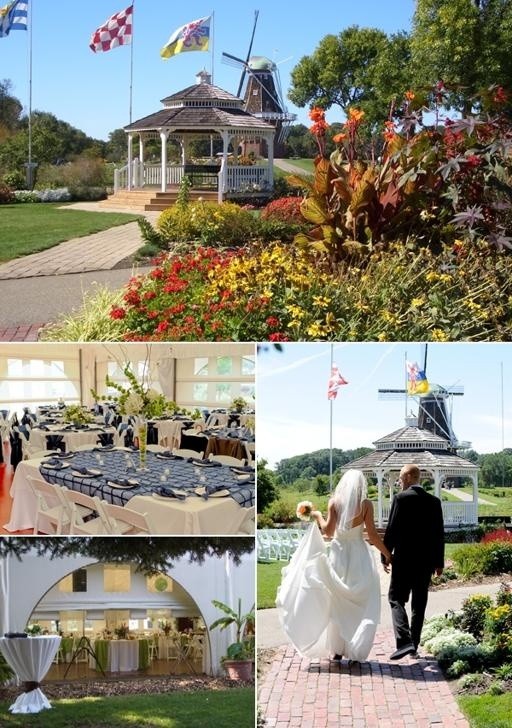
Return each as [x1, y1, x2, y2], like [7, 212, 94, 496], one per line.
[184, 163, 221, 192]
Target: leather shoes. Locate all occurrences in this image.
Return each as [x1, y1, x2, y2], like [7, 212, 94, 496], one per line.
[390, 643, 420, 660]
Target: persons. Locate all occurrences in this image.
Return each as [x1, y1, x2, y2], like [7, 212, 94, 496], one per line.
[274, 465, 445, 666]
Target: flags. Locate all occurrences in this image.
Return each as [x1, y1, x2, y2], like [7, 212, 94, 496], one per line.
[406, 360, 429, 397]
[327, 361, 348, 402]
[89, 5, 133, 54]
[0, 0, 27, 38]
[160, 14, 210, 62]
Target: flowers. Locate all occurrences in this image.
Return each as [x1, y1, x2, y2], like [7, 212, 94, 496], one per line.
[295, 499, 313, 522]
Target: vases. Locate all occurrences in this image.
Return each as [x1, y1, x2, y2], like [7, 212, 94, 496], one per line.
[209, 599, 254, 681]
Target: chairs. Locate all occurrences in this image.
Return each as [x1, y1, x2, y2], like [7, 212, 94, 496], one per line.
[257, 529, 306, 564]
[0, 627, 207, 712]
